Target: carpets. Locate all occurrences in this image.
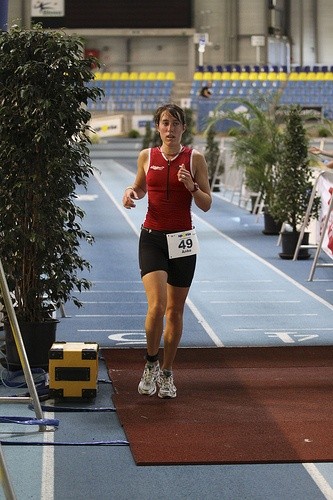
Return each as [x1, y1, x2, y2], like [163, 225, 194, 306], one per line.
[103, 345, 333, 466]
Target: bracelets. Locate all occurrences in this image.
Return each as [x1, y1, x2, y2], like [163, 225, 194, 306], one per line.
[125, 186, 134, 192]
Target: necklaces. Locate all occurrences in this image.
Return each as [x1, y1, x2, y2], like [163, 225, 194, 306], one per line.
[157, 145, 184, 167]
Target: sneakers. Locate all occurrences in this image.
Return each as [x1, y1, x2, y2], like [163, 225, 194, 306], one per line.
[138, 363, 161, 397]
[156, 369, 177, 399]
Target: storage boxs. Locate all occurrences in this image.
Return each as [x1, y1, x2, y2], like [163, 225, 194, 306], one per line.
[48, 341, 100, 405]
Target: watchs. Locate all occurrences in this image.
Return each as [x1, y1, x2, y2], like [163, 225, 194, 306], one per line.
[190, 182, 199, 193]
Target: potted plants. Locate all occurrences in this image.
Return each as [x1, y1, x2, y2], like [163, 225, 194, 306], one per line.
[238, 129, 272, 215]
[260, 129, 289, 236]
[274, 103, 322, 260]
[0, 17, 106, 370]
[204, 126, 225, 192]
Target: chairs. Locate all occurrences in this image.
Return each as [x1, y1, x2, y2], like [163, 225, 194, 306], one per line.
[84, 64, 333, 120]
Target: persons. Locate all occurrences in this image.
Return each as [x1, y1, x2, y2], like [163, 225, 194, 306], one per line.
[309, 146, 333, 168]
[198, 86, 213, 98]
[123, 104, 213, 398]
